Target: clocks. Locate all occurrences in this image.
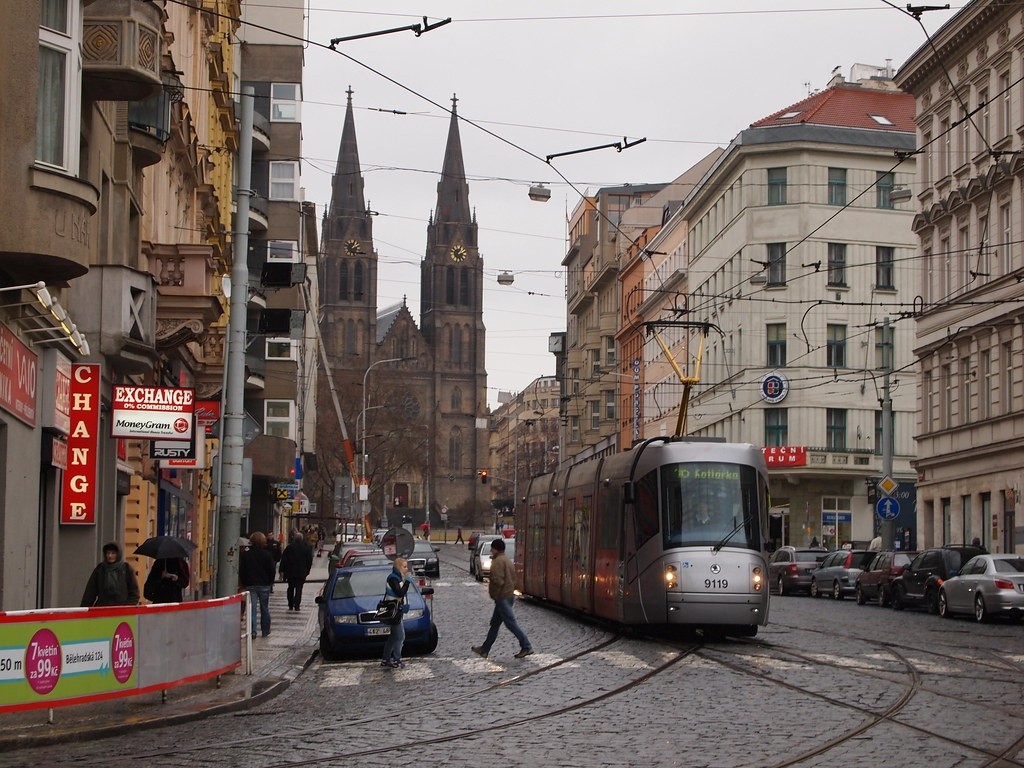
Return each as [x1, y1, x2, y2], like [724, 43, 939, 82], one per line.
[344, 239, 362, 257]
[450, 243, 468, 262]
[548, 335, 563, 352]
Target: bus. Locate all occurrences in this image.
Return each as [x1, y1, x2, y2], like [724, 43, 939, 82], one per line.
[515, 436, 773, 639]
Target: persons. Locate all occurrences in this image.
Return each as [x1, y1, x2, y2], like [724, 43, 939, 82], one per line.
[278, 533, 313, 611]
[688, 502, 716, 527]
[238, 548, 247, 620]
[290, 525, 326, 557]
[471, 539, 534, 659]
[809, 537, 819, 547]
[423, 526, 430, 541]
[266, 531, 282, 594]
[869, 532, 882, 551]
[79, 542, 141, 608]
[144, 558, 190, 604]
[496, 520, 504, 534]
[454, 528, 464, 544]
[395, 495, 403, 507]
[969, 537, 986, 552]
[380, 558, 412, 668]
[245, 532, 275, 639]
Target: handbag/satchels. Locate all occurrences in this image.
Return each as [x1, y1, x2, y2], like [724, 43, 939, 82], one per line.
[144, 583, 160, 601]
[374, 599, 404, 624]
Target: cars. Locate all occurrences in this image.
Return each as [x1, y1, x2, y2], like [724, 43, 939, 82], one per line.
[468, 529, 515, 581]
[855, 549, 922, 608]
[809, 548, 881, 600]
[314, 566, 439, 656]
[938, 554, 1024, 622]
[327, 524, 394, 579]
[408, 540, 440, 578]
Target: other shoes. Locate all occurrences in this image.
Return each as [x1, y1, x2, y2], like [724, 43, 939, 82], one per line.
[252, 635, 256, 639]
[262, 629, 271, 637]
[380, 658, 406, 670]
[289, 605, 300, 611]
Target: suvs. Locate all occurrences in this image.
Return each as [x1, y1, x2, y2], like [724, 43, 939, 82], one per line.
[890, 544, 991, 615]
[770, 545, 831, 596]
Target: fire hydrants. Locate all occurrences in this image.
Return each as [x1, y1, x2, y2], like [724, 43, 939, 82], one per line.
[482, 471, 487, 484]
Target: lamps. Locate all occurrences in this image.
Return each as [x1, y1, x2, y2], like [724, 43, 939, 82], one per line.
[0, 280, 92, 357]
[527, 181, 552, 202]
[496, 270, 514, 286]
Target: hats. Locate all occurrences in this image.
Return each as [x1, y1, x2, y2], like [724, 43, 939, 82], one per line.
[491, 537, 505, 551]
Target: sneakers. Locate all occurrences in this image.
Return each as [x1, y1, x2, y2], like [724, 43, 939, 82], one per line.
[514, 647, 534, 658]
[472, 645, 489, 658]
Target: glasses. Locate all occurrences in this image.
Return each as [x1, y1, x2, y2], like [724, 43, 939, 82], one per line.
[403, 565, 408, 568]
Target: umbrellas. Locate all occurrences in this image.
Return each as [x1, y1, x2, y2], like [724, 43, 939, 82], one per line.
[133, 536, 198, 573]
[420, 524, 430, 528]
[211, 537, 252, 547]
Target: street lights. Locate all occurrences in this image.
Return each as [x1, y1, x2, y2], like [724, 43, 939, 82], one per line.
[360, 355, 419, 536]
[356, 404, 394, 524]
